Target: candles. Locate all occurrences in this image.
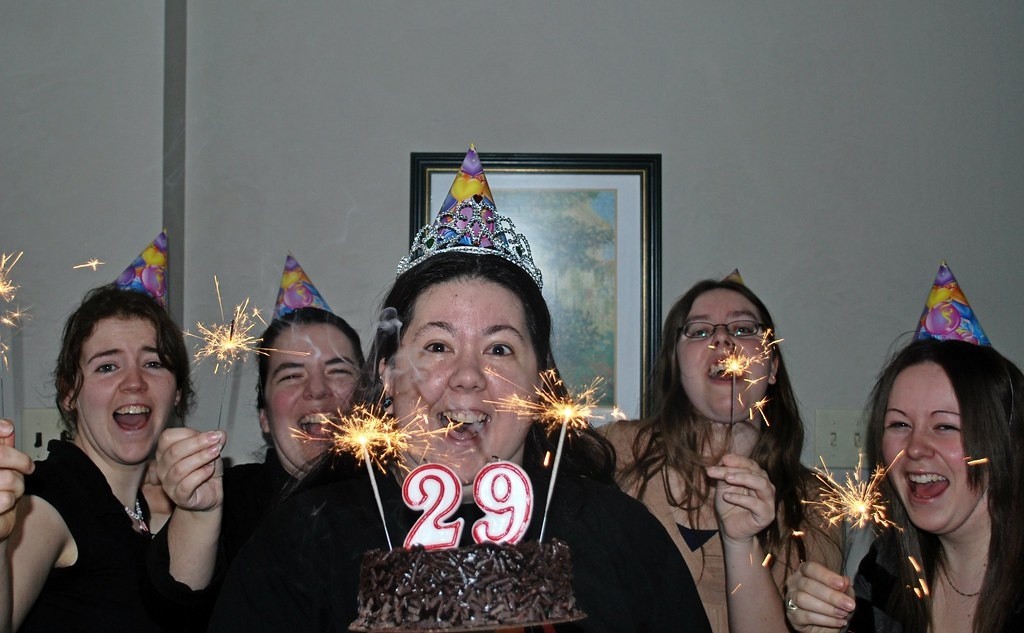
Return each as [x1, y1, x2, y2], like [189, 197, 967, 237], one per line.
[402, 463, 465, 552]
[471, 458, 533, 547]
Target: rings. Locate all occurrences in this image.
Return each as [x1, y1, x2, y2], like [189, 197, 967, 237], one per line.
[787, 597, 799, 610]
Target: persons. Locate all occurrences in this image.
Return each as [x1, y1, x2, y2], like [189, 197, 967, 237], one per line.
[222, 308, 366, 535]
[0, 284, 198, 633]
[146, 194, 716, 633]
[786, 337, 1024, 633]
[582, 276, 847, 633]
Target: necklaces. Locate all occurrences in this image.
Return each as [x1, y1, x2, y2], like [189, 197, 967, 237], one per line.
[939, 545, 980, 596]
[126, 500, 149, 532]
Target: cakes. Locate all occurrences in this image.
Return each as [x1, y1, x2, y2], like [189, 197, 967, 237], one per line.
[350, 537, 582, 631]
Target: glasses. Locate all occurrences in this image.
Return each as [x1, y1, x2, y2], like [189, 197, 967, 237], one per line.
[681, 320, 765, 339]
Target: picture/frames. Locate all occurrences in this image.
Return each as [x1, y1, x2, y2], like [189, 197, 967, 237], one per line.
[409, 152, 662, 429]
